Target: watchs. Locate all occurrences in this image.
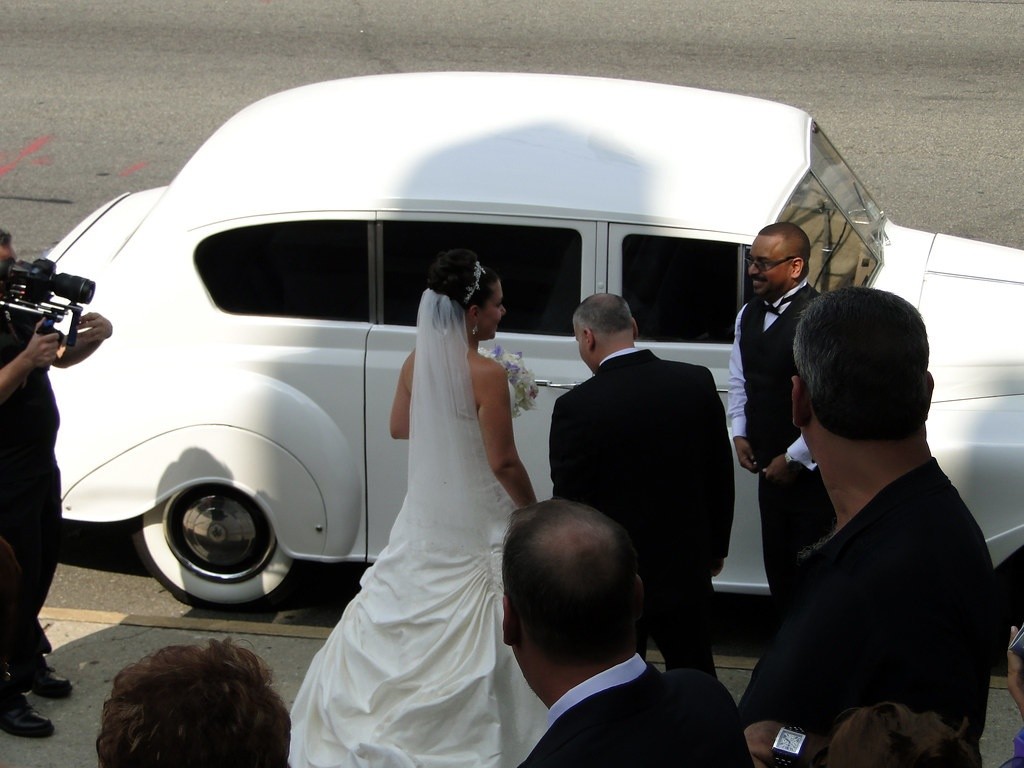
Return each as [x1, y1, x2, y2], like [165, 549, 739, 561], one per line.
[769, 719, 806, 768]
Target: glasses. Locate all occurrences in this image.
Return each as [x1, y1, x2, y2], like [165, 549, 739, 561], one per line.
[742, 256, 797, 272]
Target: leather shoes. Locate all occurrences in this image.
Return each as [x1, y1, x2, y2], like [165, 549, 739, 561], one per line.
[0, 688, 54, 738]
[10, 662, 72, 699]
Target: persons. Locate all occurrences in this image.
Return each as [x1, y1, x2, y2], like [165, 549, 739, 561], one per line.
[95, 637, 291, 768]
[726, 222, 826, 607]
[0, 227, 113, 739]
[501, 497, 754, 768]
[828, 620, 1024, 768]
[283, 248, 538, 767]
[735, 284, 1001, 767]
[548, 292, 735, 677]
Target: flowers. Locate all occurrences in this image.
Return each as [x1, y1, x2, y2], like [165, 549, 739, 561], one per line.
[485, 344, 540, 417]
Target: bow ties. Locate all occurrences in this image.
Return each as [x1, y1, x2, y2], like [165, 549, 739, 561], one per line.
[759, 286, 808, 317]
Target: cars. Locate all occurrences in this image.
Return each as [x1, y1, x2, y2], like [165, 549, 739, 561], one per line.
[29, 70, 1024, 616]
